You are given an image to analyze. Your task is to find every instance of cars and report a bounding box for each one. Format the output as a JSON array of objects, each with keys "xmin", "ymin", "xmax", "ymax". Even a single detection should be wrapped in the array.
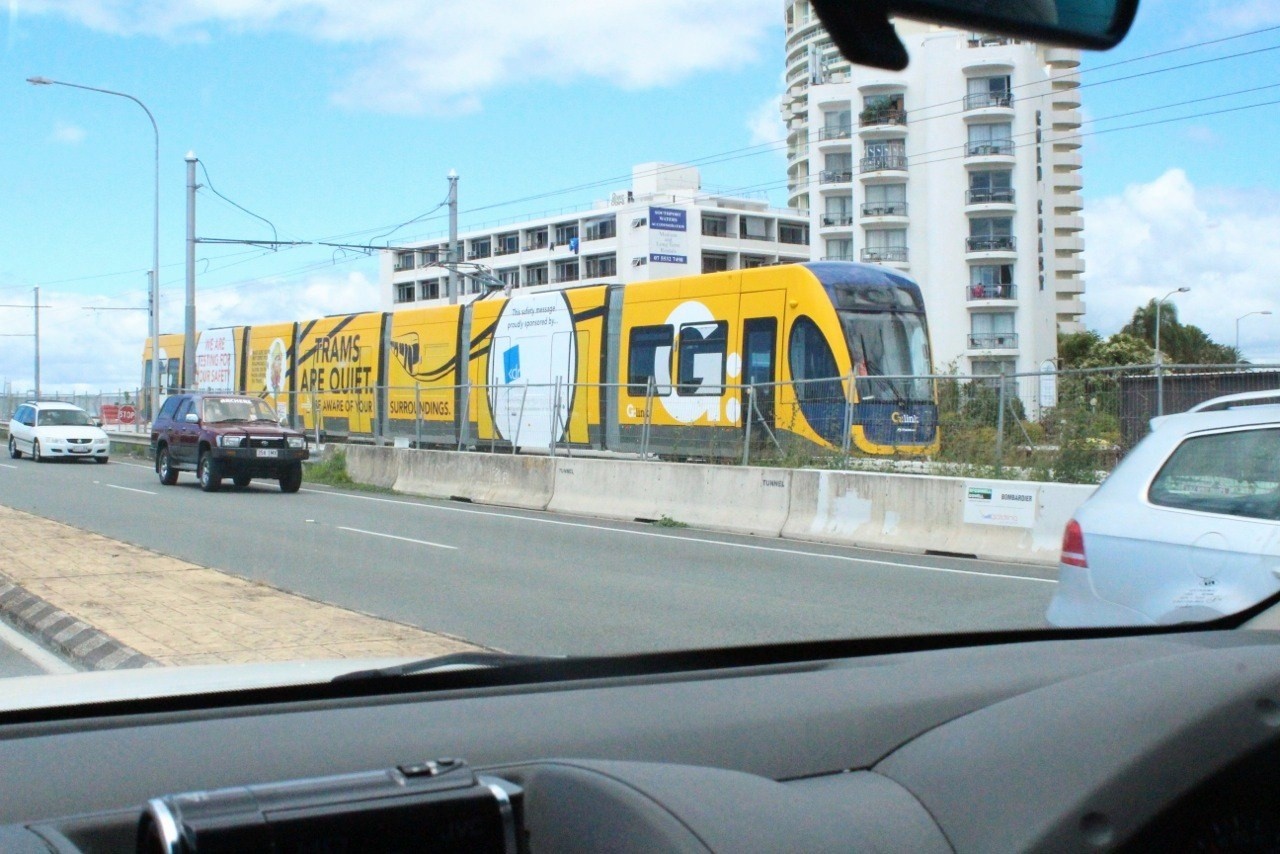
[
  {"xmin": 7, "ymin": 400, "xmax": 110, "ymax": 464},
  {"xmin": 1045, "ymin": 386, "xmax": 1280, "ymax": 627}
]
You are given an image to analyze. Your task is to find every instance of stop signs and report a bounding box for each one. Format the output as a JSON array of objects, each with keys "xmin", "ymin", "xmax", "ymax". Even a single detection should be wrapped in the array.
[{"xmin": 116, "ymin": 405, "xmax": 137, "ymax": 425}]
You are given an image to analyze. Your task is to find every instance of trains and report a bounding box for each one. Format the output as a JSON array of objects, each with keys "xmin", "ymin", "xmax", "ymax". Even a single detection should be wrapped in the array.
[{"xmin": 141, "ymin": 260, "xmax": 941, "ymax": 470}]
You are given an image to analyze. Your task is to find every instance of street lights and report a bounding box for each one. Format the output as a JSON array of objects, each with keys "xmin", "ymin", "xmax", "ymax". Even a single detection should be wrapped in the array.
[
  {"xmin": 1157, "ymin": 286, "xmax": 1191, "ymax": 416},
  {"xmin": 24, "ymin": 77, "xmax": 160, "ymax": 427},
  {"xmin": 1236, "ymin": 310, "xmax": 1273, "ymax": 372}
]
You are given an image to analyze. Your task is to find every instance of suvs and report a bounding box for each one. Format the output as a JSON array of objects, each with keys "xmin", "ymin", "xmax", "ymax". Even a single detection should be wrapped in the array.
[{"xmin": 147, "ymin": 393, "xmax": 308, "ymax": 493}]
